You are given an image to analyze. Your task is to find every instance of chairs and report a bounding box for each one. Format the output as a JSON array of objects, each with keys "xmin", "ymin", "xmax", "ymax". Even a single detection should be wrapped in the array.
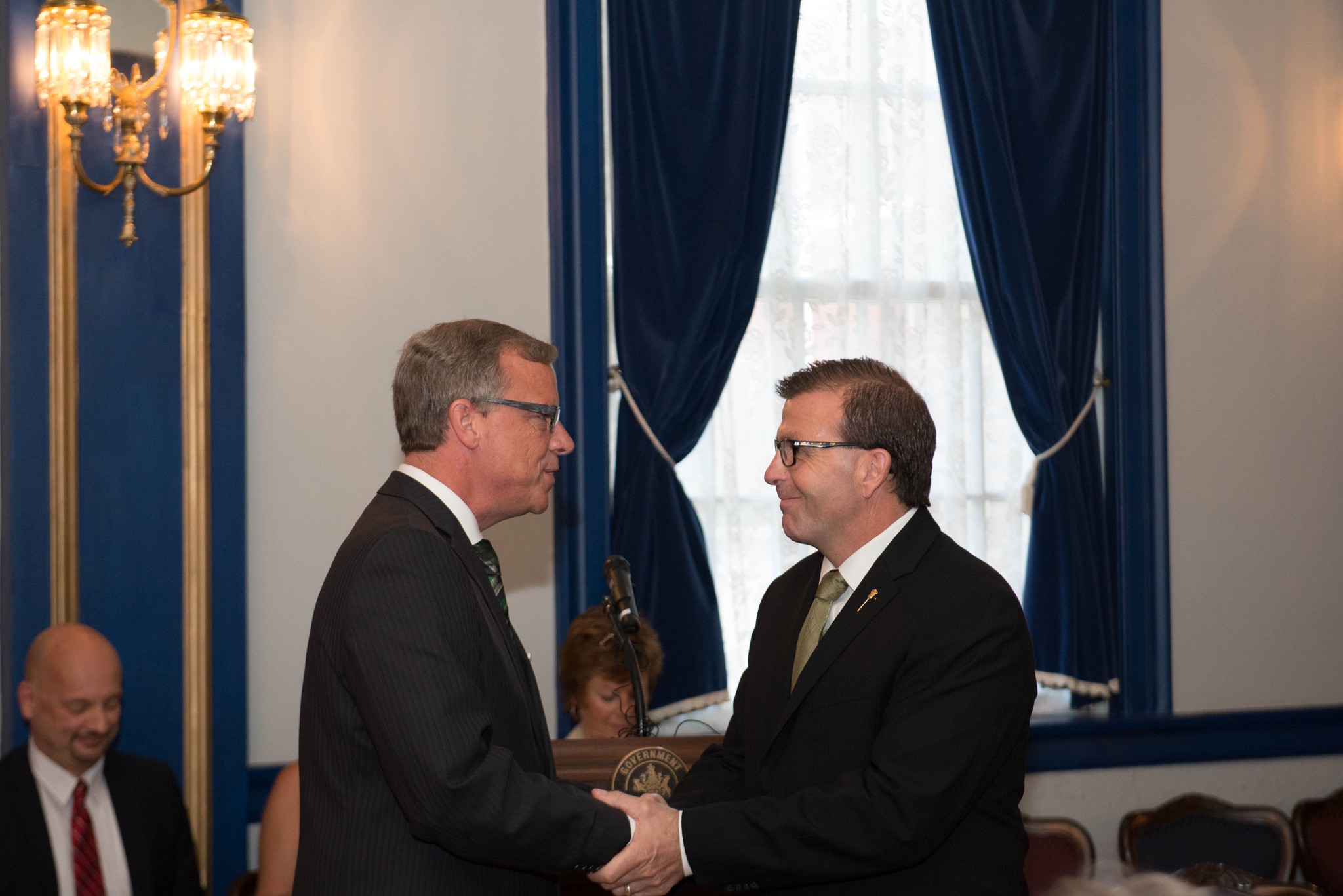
[
  {"xmin": 1289, "ymin": 787, "xmax": 1343, "ymax": 896},
  {"xmin": 1117, "ymin": 791, "xmax": 1298, "ymax": 884},
  {"xmin": 1020, "ymin": 811, "xmax": 1097, "ymax": 896}
]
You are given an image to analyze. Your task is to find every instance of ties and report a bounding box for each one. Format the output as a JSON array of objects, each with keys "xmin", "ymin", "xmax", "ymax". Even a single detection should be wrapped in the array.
[
  {"xmin": 789, "ymin": 569, "xmax": 848, "ymax": 695},
  {"xmin": 63, "ymin": 777, "xmax": 105, "ymax": 896},
  {"xmin": 474, "ymin": 539, "xmax": 509, "ymax": 621}
]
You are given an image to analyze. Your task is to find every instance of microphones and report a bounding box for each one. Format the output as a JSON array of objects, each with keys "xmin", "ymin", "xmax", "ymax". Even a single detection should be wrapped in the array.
[{"xmin": 605, "ymin": 555, "xmax": 642, "ymax": 635}]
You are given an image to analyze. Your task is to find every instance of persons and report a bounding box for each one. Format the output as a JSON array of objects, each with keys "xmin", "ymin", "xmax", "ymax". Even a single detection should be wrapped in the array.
[
  {"xmin": 289, "ymin": 318, "xmax": 659, "ymax": 896},
  {"xmin": 587, "ymin": 352, "xmax": 1037, "ymax": 896},
  {"xmin": 0, "ymin": 623, "xmax": 202, "ymax": 896},
  {"xmin": 559, "ymin": 601, "xmax": 663, "ymax": 738},
  {"xmin": 257, "ymin": 760, "xmax": 300, "ymax": 896}
]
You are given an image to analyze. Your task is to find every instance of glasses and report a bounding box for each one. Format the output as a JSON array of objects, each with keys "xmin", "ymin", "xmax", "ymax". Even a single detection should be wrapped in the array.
[
  {"xmin": 774, "ymin": 438, "xmax": 898, "ymax": 474},
  {"xmin": 469, "ymin": 397, "xmax": 561, "ymax": 434}
]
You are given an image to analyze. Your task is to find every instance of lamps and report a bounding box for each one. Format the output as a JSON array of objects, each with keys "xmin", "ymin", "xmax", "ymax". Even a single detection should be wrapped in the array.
[{"xmin": 31, "ymin": 0, "xmax": 262, "ymax": 247}]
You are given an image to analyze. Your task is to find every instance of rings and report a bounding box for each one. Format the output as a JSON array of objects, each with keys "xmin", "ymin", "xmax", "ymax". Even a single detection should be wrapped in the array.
[{"xmin": 625, "ymin": 883, "xmax": 631, "ymax": 896}]
